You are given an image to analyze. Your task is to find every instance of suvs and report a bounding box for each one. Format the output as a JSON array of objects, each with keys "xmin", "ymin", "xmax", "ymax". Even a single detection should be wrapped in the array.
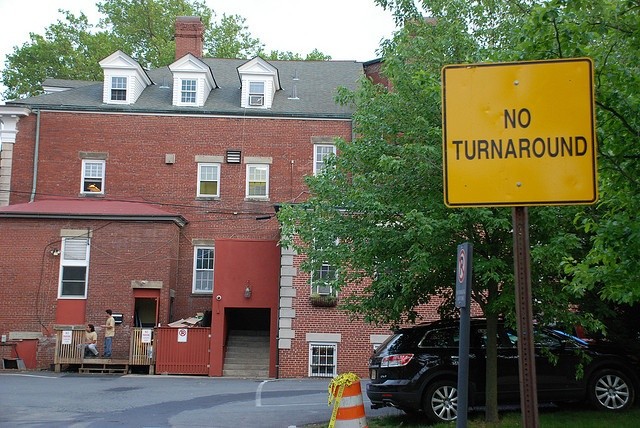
[{"xmin": 367, "ymin": 316, "xmax": 640, "ymax": 423}]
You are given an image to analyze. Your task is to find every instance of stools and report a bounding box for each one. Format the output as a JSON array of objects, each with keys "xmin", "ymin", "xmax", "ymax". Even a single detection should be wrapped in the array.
[{"xmin": 85, "ymin": 346, "xmax": 97, "ymax": 357}]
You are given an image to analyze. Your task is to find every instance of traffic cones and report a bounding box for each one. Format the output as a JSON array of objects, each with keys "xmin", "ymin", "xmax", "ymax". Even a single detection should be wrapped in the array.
[{"xmin": 332, "ymin": 380, "xmax": 369, "ymax": 428}]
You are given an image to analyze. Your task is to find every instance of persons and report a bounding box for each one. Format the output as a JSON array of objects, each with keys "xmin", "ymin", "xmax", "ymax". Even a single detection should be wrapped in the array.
[
  {"xmin": 100, "ymin": 308, "xmax": 115, "ymax": 358},
  {"xmin": 83, "ymin": 323, "xmax": 99, "ymax": 357}
]
